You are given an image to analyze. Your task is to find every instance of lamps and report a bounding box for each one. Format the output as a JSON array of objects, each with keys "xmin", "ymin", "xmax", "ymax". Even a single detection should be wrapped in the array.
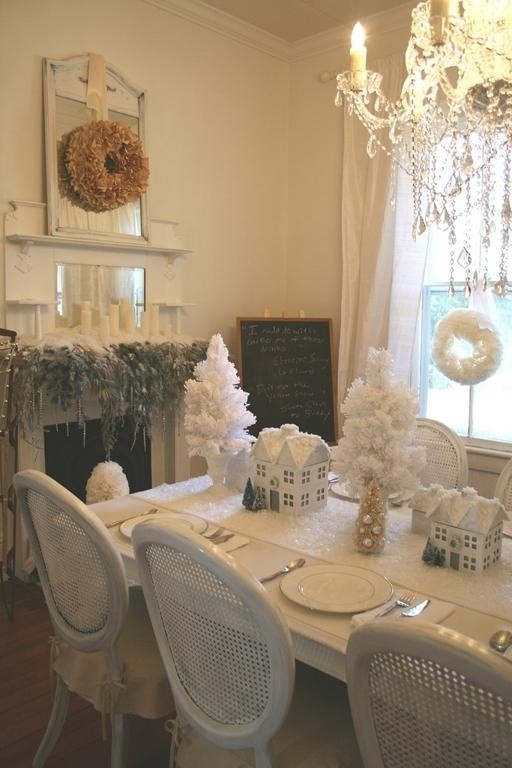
[{"xmin": 336, "ymin": 2, "xmax": 512, "ymax": 300}]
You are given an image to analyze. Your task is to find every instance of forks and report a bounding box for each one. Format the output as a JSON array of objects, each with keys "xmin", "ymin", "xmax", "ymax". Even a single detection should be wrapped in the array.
[
  {"xmin": 374, "ymin": 591, "xmax": 417, "ymax": 619},
  {"xmin": 202, "ymin": 526, "xmax": 226, "ymax": 539}
]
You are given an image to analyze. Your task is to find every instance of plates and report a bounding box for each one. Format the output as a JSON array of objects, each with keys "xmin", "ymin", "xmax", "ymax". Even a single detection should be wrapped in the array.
[
  {"xmin": 118, "ymin": 511, "xmax": 209, "ymax": 543},
  {"xmin": 279, "ymin": 563, "xmax": 394, "ymax": 614},
  {"xmin": 331, "ymin": 479, "xmax": 401, "ymax": 500}
]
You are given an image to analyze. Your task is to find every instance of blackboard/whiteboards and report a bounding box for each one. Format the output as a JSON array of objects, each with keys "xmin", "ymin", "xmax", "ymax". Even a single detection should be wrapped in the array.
[{"xmin": 237, "ymin": 317, "xmax": 338, "ymax": 446}]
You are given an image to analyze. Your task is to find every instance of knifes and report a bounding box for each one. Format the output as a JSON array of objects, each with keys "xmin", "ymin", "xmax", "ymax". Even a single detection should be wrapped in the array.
[
  {"xmin": 395, "ymin": 599, "xmax": 430, "ymax": 618},
  {"xmin": 209, "ymin": 534, "xmax": 235, "ymax": 545}
]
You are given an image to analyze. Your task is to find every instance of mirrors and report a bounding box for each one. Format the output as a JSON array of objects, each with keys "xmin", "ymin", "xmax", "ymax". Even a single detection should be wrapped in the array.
[
  {"xmin": 42, "ymin": 54, "xmax": 152, "ymax": 241},
  {"xmin": 56, "ymin": 262, "xmax": 145, "ymax": 329}
]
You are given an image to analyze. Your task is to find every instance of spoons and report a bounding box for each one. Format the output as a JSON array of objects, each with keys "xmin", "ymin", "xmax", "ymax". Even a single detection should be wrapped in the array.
[
  {"xmin": 489, "ymin": 629, "xmax": 512, "ymax": 654},
  {"xmin": 390, "ymin": 495, "xmax": 412, "ymax": 507},
  {"xmin": 257, "ymin": 558, "xmax": 306, "ymax": 585},
  {"xmin": 104, "ymin": 508, "xmax": 158, "ymax": 529}
]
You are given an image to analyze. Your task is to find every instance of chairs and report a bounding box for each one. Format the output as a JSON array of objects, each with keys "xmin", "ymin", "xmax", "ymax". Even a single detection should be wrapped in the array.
[
  {"xmin": 491, "ymin": 454, "xmax": 511, "ymax": 510},
  {"xmin": 414, "ymin": 419, "xmax": 469, "ymax": 492},
  {"xmin": 125, "ymin": 515, "xmax": 296, "ymax": 766},
  {"xmin": 12, "ymin": 467, "xmax": 173, "ymax": 765},
  {"xmin": 335, "ymin": 616, "xmax": 512, "ymax": 767}
]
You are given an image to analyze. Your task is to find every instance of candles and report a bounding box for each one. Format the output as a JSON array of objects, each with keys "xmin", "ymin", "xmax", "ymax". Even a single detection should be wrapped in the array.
[{"xmin": 78, "ymin": 297, "xmax": 182, "ymax": 343}]
